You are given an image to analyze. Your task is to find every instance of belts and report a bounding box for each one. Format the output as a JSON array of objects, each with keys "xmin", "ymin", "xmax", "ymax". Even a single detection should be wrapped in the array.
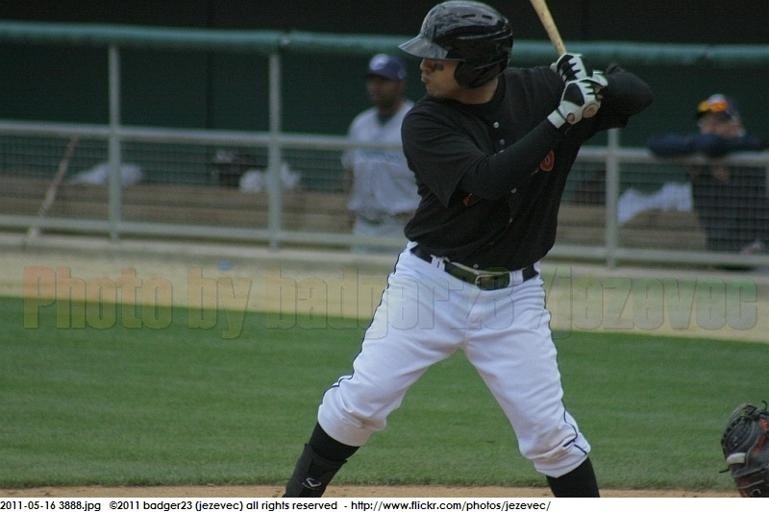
[{"xmin": 410, "ymin": 241, "xmax": 539, "ymax": 290}]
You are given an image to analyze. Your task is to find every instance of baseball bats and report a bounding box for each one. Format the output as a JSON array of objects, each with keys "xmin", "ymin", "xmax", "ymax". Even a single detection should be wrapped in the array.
[{"xmin": 532, "ymin": 1, "xmax": 599, "ymax": 118}]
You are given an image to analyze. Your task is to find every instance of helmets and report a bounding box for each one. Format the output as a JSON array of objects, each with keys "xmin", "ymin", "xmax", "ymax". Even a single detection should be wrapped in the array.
[{"xmin": 398, "ymin": 0, "xmax": 514, "ymax": 90}]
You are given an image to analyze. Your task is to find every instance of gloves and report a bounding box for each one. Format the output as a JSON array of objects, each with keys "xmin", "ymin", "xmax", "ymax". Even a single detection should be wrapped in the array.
[{"xmin": 546, "ymin": 51, "xmax": 609, "ymax": 135}]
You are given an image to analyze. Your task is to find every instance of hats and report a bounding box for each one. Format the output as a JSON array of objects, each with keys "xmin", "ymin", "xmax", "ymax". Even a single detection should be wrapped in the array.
[{"xmin": 364, "ymin": 53, "xmax": 405, "ymax": 80}]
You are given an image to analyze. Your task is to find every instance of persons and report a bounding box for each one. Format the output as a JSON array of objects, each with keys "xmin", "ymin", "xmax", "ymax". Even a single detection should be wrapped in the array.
[
  {"xmin": 646, "ymin": 94, "xmax": 769, "ymax": 272},
  {"xmin": 281, "ymin": 0, "xmax": 657, "ymax": 497},
  {"xmin": 341, "ymin": 52, "xmax": 422, "ymax": 257}
]
